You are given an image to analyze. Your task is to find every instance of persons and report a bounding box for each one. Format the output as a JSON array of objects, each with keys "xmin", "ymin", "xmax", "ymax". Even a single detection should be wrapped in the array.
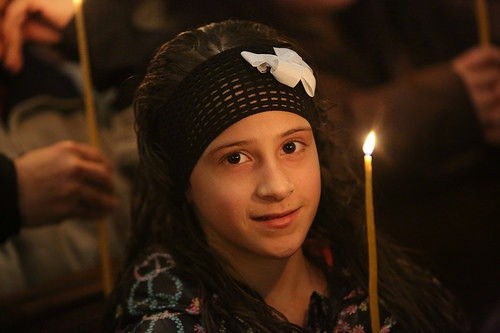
[
  {"xmin": 110, "ymin": 17, "xmax": 466, "ymax": 333},
  {"xmin": 277, "ymin": 1, "xmax": 500, "ymax": 332}
]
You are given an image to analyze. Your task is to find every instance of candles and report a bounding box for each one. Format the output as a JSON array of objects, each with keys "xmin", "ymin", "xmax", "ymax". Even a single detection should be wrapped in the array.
[
  {"xmin": 70, "ymin": 0, "xmax": 118, "ymax": 296},
  {"xmin": 361, "ymin": 128, "xmax": 383, "ymax": 332}
]
